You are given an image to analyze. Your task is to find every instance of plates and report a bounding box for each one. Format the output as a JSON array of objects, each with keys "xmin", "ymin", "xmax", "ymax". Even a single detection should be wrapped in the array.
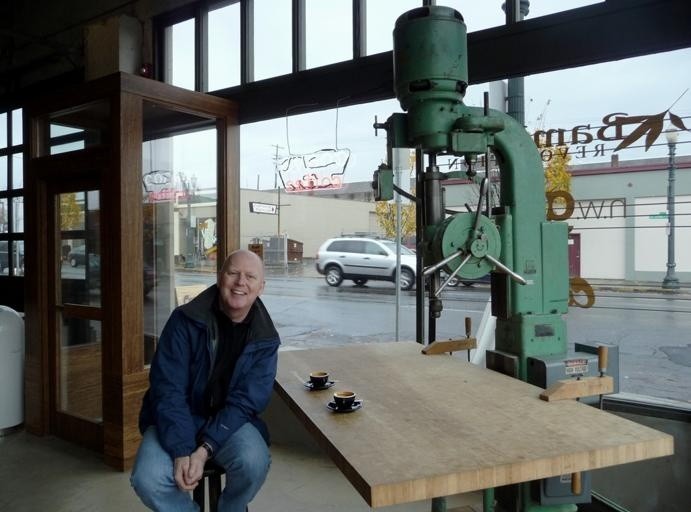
[
  {"xmin": 303, "ymin": 379, "xmax": 336, "ymax": 390},
  {"xmin": 327, "ymin": 398, "xmax": 363, "ymax": 414}
]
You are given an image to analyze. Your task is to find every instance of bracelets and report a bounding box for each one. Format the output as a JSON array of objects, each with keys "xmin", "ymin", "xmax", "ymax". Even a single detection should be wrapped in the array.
[{"xmin": 200, "ymin": 438, "xmax": 213, "ymax": 460}]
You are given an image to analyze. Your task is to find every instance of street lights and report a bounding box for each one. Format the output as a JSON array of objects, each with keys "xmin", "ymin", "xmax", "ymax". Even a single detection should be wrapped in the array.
[{"xmin": 663, "ymin": 123, "xmax": 681, "ymax": 289}]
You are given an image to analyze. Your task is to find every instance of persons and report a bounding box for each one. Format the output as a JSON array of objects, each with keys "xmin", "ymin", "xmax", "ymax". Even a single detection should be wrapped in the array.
[{"xmin": 129, "ymin": 247, "xmax": 280, "ymax": 512}]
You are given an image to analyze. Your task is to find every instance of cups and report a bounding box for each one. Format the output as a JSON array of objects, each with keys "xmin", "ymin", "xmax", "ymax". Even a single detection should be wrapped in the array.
[
  {"xmin": 334, "ymin": 390, "xmax": 356, "ymax": 409},
  {"xmin": 310, "ymin": 370, "xmax": 330, "ymax": 387}
]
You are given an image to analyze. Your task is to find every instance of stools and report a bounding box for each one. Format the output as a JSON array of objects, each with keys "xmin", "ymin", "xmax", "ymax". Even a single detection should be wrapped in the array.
[{"xmin": 192, "ymin": 460, "xmax": 227, "ymax": 511}]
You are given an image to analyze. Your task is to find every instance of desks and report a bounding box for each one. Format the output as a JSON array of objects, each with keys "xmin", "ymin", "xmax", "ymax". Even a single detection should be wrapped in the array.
[{"xmin": 273, "ymin": 341, "xmax": 675, "ymax": 507}]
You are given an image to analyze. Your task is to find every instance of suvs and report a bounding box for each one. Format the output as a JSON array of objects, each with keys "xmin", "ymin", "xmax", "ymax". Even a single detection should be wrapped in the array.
[{"xmin": 316, "ymin": 237, "xmax": 417, "ymax": 290}]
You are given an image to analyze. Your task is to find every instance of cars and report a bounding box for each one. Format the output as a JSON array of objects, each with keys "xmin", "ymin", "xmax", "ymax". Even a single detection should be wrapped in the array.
[{"xmin": 67, "ymin": 245, "xmax": 86, "ymax": 267}]
